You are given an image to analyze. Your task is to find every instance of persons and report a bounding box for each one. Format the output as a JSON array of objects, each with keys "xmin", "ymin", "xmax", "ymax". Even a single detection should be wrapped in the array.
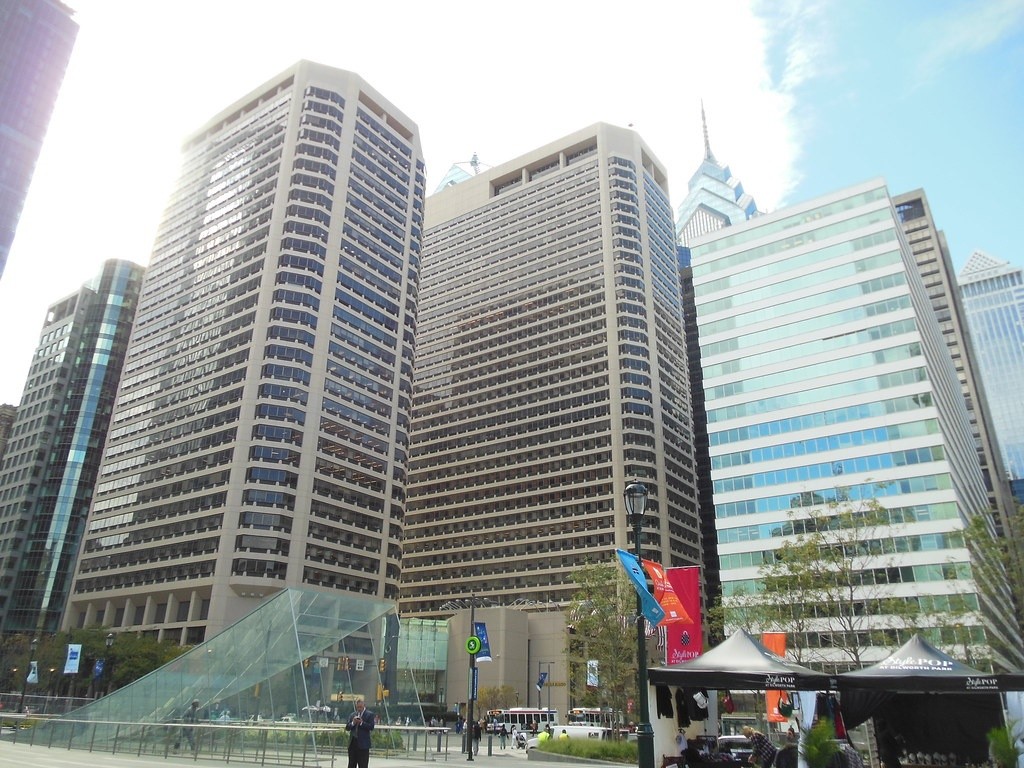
[
  {"xmin": 742, "ymin": 725, "xmax": 777, "ymax": 768},
  {"xmin": 373, "ymin": 713, "xmax": 449, "ymax": 734},
  {"xmin": 455, "ymin": 714, "xmax": 571, "ymax": 756},
  {"xmin": 345, "ymin": 699, "xmax": 375, "ymax": 768},
  {"xmin": 247, "ymin": 715, "xmax": 266, "ymax": 726},
  {"xmin": 175, "ymin": 699, "xmax": 199, "ymax": 756},
  {"xmin": 220, "ymin": 710, "xmax": 230, "ymax": 725}
]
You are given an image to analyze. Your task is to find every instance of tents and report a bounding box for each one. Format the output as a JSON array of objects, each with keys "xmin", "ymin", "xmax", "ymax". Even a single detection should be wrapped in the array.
[
  {"xmin": 837, "ymin": 633, "xmax": 1024, "ymax": 768},
  {"xmin": 648, "ymin": 628, "xmax": 834, "ymax": 768}
]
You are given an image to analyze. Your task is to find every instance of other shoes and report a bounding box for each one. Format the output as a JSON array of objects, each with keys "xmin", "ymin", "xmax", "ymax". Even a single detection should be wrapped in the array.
[
  {"xmin": 173, "ymin": 748, "xmax": 179, "ymax": 754},
  {"xmin": 190, "ymin": 750, "xmax": 197, "ymax": 755}
]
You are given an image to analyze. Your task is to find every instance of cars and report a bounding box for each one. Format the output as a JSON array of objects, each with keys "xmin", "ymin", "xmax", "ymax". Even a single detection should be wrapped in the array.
[{"xmin": 718, "ymin": 735, "xmax": 782, "ymax": 753}]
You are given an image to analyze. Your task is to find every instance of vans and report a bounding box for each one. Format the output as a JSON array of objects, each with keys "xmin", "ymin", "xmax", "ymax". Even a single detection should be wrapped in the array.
[{"xmin": 525, "ymin": 726, "xmax": 629, "ymax": 757}]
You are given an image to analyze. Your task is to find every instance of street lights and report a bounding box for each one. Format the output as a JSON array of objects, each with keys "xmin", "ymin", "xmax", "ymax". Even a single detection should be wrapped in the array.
[
  {"xmin": 466, "ymin": 594, "xmax": 485, "ymax": 761},
  {"xmin": 624, "ymin": 478, "xmax": 655, "ymax": 768},
  {"xmin": 204, "ymin": 649, "xmax": 212, "ymax": 719},
  {"xmin": 42, "ymin": 669, "xmax": 55, "ymax": 714},
  {"xmin": 103, "ymin": 633, "xmax": 115, "ymax": 696},
  {"xmin": 538, "ymin": 660, "xmax": 555, "ymax": 707},
  {"xmin": 10, "ymin": 638, "xmax": 39, "ymax": 731},
  {"xmin": 5, "ymin": 669, "xmax": 17, "ymax": 712},
  {"xmin": 440, "ymin": 688, "xmax": 443, "ymax": 704}
]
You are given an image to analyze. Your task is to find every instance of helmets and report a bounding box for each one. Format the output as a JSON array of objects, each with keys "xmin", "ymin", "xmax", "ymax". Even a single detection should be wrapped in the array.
[{"xmin": 191, "ymin": 699, "xmax": 199, "ymax": 705}]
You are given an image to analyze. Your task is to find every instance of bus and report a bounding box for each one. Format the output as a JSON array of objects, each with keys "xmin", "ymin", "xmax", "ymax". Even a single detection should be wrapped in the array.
[
  {"xmin": 484, "ymin": 707, "xmax": 559, "ymax": 734},
  {"xmin": 565, "ymin": 707, "xmax": 624, "ymax": 728}
]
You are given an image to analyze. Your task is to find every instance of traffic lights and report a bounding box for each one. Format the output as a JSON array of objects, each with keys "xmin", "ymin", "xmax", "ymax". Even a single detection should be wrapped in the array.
[
  {"xmin": 304, "ymin": 659, "xmax": 309, "ymax": 669},
  {"xmin": 380, "ymin": 660, "xmax": 385, "ymax": 672},
  {"xmin": 337, "ymin": 657, "xmax": 349, "ymax": 671}
]
